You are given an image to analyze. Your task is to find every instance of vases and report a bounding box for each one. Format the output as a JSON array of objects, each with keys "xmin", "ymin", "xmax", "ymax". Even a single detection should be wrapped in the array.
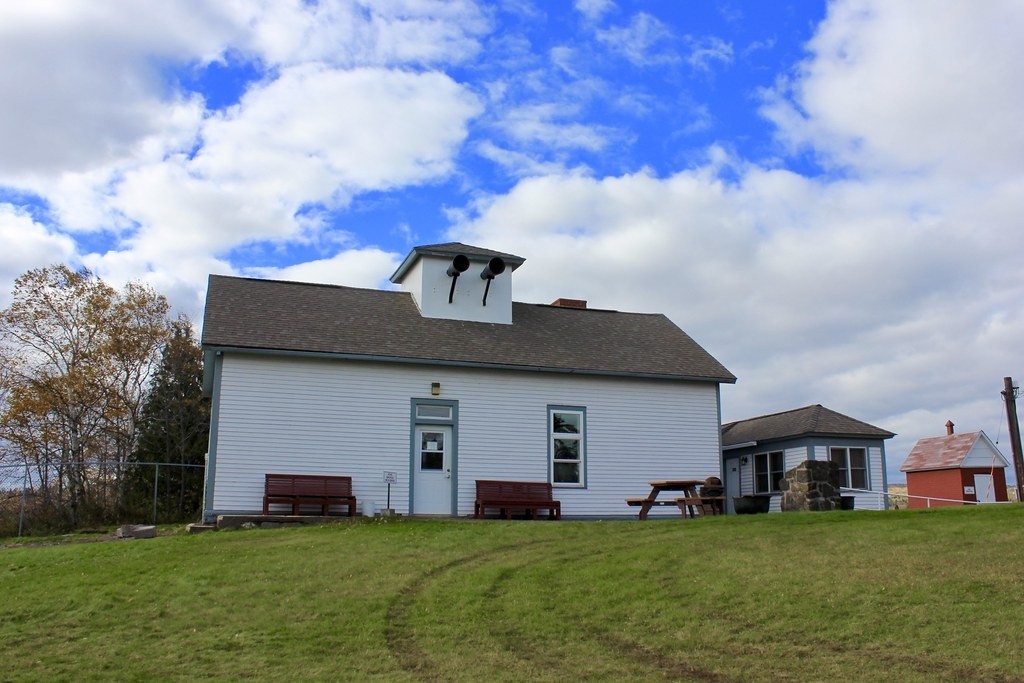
[
  {"xmin": 732, "ymin": 494, "xmax": 773, "ymax": 514},
  {"xmin": 841, "ymin": 496, "xmax": 856, "ymax": 510}
]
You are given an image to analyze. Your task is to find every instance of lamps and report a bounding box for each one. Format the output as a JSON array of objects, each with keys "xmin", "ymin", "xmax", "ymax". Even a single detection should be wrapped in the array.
[
  {"xmin": 742, "ymin": 456, "xmax": 748, "ymax": 465},
  {"xmin": 432, "ymin": 383, "xmax": 440, "ymax": 395}
]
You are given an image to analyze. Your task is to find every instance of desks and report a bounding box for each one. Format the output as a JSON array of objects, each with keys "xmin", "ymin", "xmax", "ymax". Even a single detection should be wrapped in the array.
[{"xmin": 639, "ymin": 481, "xmax": 723, "ymax": 520}]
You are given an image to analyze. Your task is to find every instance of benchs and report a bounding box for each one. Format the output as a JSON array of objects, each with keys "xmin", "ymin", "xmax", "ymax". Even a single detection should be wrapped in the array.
[
  {"xmin": 263, "ymin": 474, "xmax": 356, "ymax": 517},
  {"xmin": 475, "ymin": 480, "xmax": 561, "ymax": 520},
  {"xmin": 674, "ymin": 496, "xmax": 727, "ymax": 506},
  {"xmin": 625, "ymin": 498, "xmax": 685, "ymax": 506}
]
